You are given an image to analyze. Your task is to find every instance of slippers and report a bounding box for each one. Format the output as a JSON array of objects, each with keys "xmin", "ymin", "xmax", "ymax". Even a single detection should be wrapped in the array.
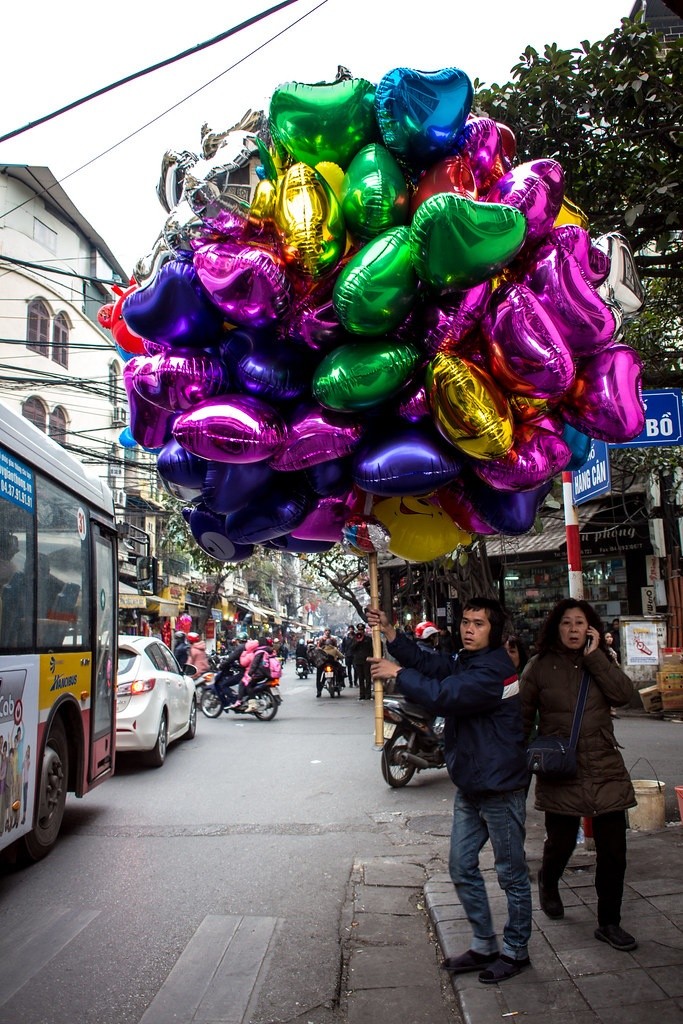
[
  {"xmin": 439, "ymin": 949, "xmax": 501, "ymax": 971},
  {"xmin": 478, "ymin": 956, "xmax": 533, "ymax": 983}
]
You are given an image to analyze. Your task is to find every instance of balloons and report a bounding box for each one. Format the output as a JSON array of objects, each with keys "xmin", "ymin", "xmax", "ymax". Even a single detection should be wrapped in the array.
[{"xmin": 97, "ymin": 66, "xmax": 651, "ymax": 562}]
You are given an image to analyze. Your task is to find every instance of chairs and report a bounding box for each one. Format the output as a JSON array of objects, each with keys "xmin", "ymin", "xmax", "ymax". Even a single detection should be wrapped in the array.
[
  {"xmin": 38, "ymin": 580, "xmax": 80, "ymax": 650},
  {"xmin": 0, "ymin": 573, "xmax": 28, "ymax": 654}
]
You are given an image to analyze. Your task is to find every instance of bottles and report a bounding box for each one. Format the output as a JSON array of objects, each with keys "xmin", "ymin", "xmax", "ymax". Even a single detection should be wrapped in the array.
[{"xmin": 503, "ymin": 560, "xmax": 618, "ymax": 619}]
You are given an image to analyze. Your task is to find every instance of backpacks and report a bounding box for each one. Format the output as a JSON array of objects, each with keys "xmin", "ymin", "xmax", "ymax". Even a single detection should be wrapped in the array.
[{"xmin": 264, "ymin": 652, "xmax": 281, "ymax": 680}]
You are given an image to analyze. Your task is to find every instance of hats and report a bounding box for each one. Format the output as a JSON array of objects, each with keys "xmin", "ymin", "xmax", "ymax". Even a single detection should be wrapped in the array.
[
  {"xmin": 273, "ymin": 637, "xmax": 281, "ymax": 643},
  {"xmin": 299, "ymin": 639, "xmax": 304, "ymax": 645},
  {"xmin": 356, "ymin": 623, "xmax": 366, "ymax": 630}
]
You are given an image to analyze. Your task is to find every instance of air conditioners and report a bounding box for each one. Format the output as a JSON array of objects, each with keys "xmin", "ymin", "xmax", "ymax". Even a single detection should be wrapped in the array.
[
  {"xmin": 113, "ymin": 406, "xmax": 130, "ymax": 424},
  {"xmin": 114, "ymin": 489, "xmax": 127, "ymax": 506}
]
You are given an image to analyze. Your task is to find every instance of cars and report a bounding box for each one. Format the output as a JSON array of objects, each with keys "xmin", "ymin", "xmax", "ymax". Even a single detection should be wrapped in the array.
[{"xmin": 62, "ymin": 634, "xmax": 198, "ymax": 768}]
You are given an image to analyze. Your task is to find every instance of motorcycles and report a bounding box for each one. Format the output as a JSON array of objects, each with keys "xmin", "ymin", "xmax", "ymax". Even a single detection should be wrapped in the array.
[{"xmin": 168, "ymin": 645, "xmax": 286, "ymax": 722}]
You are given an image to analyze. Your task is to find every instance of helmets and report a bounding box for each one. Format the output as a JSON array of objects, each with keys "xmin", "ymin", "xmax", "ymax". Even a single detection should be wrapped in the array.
[
  {"xmin": 174, "ymin": 631, "xmax": 186, "ymax": 642},
  {"xmin": 186, "ymin": 631, "xmax": 201, "ymax": 643},
  {"xmin": 414, "ymin": 621, "xmax": 441, "ymax": 640},
  {"xmin": 236, "ymin": 631, "xmax": 249, "ymax": 641}
]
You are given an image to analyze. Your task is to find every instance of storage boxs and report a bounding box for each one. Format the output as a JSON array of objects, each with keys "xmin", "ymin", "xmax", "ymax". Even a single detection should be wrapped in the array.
[{"xmin": 639, "ymin": 648, "xmax": 683, "ymax": 714}]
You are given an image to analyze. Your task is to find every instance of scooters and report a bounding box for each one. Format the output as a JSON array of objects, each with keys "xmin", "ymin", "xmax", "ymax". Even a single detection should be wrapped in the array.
[
  {"xmin": 295, "ymin": 656, "xmax": 314, "ymax": 680},
  {"xmin": 373, "ymin": 694, "xmax": 447, "ymax": 789},
  {"xmin": 319, "ymin": 656, "xmax": 346, "ymax": 698}
]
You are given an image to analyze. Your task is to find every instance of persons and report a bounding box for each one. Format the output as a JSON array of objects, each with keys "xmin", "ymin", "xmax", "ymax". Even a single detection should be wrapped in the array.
[
  {"xmin": 186, "ymin": 631, "xmax": 211, "ymax": 679},
  {"xmin": 18, "ymin": 550, "xmax": 78, "ymax": 617},
  {"xmin": 306, "ymin": 639, "xmax": 317, "ymax": 653},
  {"xmin": 315, "ymin": 627, "xmax": 345, "ymax": 698},
  {"xmin": 216, "ymin": 631, "xmax": 274, "ymax": 712},
  {"xmin": 0, "ymin": 526, "xmax": 24, "ymax": 650},
  {"xmin": 296, "ymin": 638, "xmax": 311, "ymax": 670},
  {"xmin": 501, "ymin": 634, "xmax": 533, "ymax": 859},
  {"xmin": 603, "ymin": 631, "xmax": 620, "ymax": 666},
  {"xmin": 365, "ymin": 598, "xmax": 532, "ymax": 983},
  {"xmin": 274, "ymin": 637, "xmax": 288, "ymax": 669},
  {"xmin": 341, "ymin": 623, "xmax": 383, "ymax": 687},
  {"xmin": 414, "ymin": 622, "xmax": 445, "ymax": 745},
  {"xmin": 173, "ymin": 631, "xmax": 190, "ymax": 668},
  {"xmin": 611, "ymin": 619, "xmax": 621, "ymax": 652},
  {"xmin": 518, "ymin": 597, "xmax": 639, "ymax": 950},
  {"xmin": 437, "ymin": 622, "xmax": 456, "ymax": 654},
  {"xmin": 403, "ymin": 624, "xmax": 413, "ymax": 640}
]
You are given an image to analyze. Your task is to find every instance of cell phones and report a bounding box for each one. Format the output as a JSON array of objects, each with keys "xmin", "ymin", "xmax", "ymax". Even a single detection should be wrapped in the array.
[{"xmin": 587, "ymin": 636, "xmax": 592, "ymax": 648}]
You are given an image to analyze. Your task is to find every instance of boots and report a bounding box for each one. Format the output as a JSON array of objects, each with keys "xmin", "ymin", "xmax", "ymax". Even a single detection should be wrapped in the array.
[{"xmin": 244, "ymin": 698, "xmax": 258, "ymax": 713}]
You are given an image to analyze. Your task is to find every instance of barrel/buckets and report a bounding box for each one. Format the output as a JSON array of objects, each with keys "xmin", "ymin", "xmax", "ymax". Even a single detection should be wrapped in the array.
[
  {"xmin": 627, "ymin": 757, "xmax": 667, "ymax": 834},
  {"xmin": 674, "ymin": 786, "xmax": 683, "ymax": 825},
  {"xmin": 660, "ymin": 647, "xmax": 683, "ymax": 672}
]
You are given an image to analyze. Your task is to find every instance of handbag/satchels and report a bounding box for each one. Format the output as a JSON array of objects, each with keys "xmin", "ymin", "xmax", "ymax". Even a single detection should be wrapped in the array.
[{"xmin": 524, "ymin": 739, "xmax": 576, "ymax": 780}]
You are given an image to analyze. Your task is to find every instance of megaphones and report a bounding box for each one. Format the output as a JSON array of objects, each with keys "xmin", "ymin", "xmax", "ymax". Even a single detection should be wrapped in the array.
[{"xmin": 355, "ymin": 632, "xmax": 364, "ymax": 641}]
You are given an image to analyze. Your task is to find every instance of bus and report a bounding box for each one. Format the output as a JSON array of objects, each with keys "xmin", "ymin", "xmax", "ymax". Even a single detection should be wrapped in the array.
[{"xmin": 0, "ymin": 400, "xmax": 158, "ymax": 868}]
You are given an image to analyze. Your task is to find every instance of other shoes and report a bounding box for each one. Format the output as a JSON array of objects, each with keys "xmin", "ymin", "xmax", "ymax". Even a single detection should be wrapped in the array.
[
  {"xmin": 537, "ymin": 868, "xmax": 564, "ymax": 919},
  {"xmin": 222, "ymin": 702, "xmax": 235, "ymax": 709},
  {"xmin": 594, "ymin": 922, "xmax": 638, "ymax": 952},
  {"xmin": 231, "ymin": 699, "xmax": 243, "ymax": 708}
]
